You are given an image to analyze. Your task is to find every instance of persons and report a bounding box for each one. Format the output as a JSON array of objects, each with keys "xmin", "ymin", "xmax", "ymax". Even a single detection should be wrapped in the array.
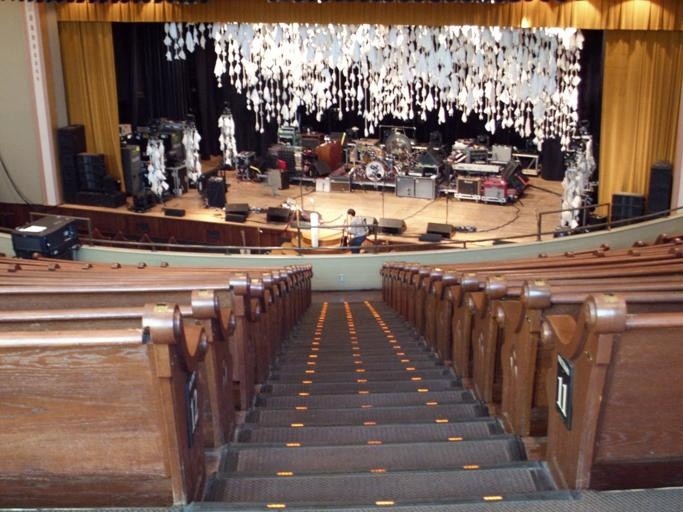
[{"xmin": 347, "ymin": 209, "xmax": 369, "ymax": 254}]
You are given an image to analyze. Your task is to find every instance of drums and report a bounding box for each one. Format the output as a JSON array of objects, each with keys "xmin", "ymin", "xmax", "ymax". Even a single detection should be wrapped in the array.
[{"xmin": 364, "ymin": 160, "xmax": 386, "ymax": 182}]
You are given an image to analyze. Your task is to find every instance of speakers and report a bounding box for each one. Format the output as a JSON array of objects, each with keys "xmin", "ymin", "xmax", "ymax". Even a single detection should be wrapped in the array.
[
  {"xmin": 208, "ymin": 177, "xmax": 227, "ymax": 208},
  {"xmin": 612, "ymin": 193, "xmax": 645, "ymax": 229},
  {"xmin": 647, "ymin": 161, "xmax": 674, "ymax": 222},
  {"xmin": 77, "ymin": 152, "xmax": 106, "ymax": 193},
  {"xmin": 55, "ymin": 123, "xmax": 87, "ymax": 189}
]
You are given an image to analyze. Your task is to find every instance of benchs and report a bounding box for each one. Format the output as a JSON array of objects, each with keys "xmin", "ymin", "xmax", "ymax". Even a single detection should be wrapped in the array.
[
  {"xmin": 306, "ymin": 265, "xmax": 311, "ymax": 307},
  {"xmin": 432, "ymin": 275, "xmax": 683, "ymax": 379},
  {"xmin": 279, "ymin": 270, "xmax": 291, "ymax": 339},
  {"xmin": 290, "ymin": 265, "xmax": 301, "ymax": 320},
  {"xmin": 467, "ymin": 277, "xmax": 682, "ymax": 402},
  {"xmin": 380, "ymin": 263, "xmax": 386, "ymax": 301},
  {"xmin": 387, "ymin": 262, "xmax": 393, "ymax": 306},
  {"xmin": 494, "ymin": 278, "xmax": 682, "ymax": 437},
  {"xmin": 0, "ymin": 304, "xmax": 209, "ymax": 507},
  {"xmin": 412, "ymin": 266, "xmax": 429, "ymax": 337},
  {"xmin": 303, "ymin": 265, "xmax": 308, "ymax": 311},
  {"xmin": 423, "ymin": 268, "xmax": 444, "ymax": 352},
  {"xmin": 391, "ymin": 264, "xmax": 398, "ymax": 310},
  {"xmin": 406, "ymin": 263, "xmax": 419, "ymax": 327},
  {"xmin": 439, "ymin": 273, "xmax": 457, "ymax": 362},
  {"xmin": 539, "ymin": 293, "xmax": 683, "ymax": 490},
  {"xmin": 394, "ymin": 263, "xmax": 404, "ymax": 314},
  {"xmin": 0, "ymin": 280, "xmax": 271, "ymax": 383},
  {"xmin": 309, "ymin": 265, "xmax": 313, "ymax": 304},
  {"xmin": 399, "ymin": 265, "xmax": 412, "ymax": 321},
  {"xmin": 0, "ymin": 290, "xmax": 235, "ymax": 448},
  {"xmin": 385, "ymin": 263, "xmax": 390, "ymax": 302},
  {"xmin": 300, "ymin": 266, "xmax": 306, "ymax": 312},
  {"xmin": 0, "ymin": 272, "xmax": 261, "ymax": 410},
  {"xmin": 285, "ymin": 267, "xmax": 297, "ymax": 326},
  {"xmin": 295, "ymin": 265, "xmax": 302, "ymax": 313}
]
